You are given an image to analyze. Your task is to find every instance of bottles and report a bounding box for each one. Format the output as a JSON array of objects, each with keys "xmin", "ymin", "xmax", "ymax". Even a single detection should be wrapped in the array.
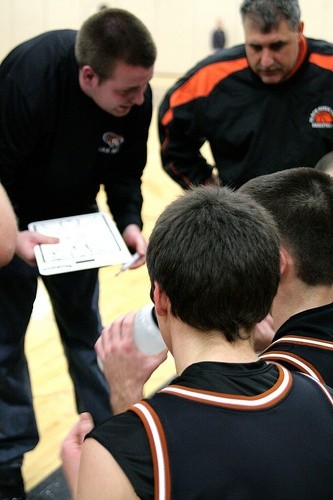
[{"xmin": 96, "ymin": 303, "xmax": 168, "ymax": 374}]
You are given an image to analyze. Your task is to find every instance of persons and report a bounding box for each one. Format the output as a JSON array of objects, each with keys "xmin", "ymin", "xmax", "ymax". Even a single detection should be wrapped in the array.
[
  {"xmin": 0, "ymin": 183, "xmax": 17, "ymax": 266},
  {"xmin": 61, "ymin": 184, "xmax": 333, "ymax": 500},
  {"xmin": 157, "ymin": 0, "xmax": 333, "ymax": 194},
  {"xmin": 95, "ymin": 166, "xmax": 333, "ymax": 416},
  {"xmin": 0, "ymin": 9, "xmax": 157, "ymax": 500}
]
